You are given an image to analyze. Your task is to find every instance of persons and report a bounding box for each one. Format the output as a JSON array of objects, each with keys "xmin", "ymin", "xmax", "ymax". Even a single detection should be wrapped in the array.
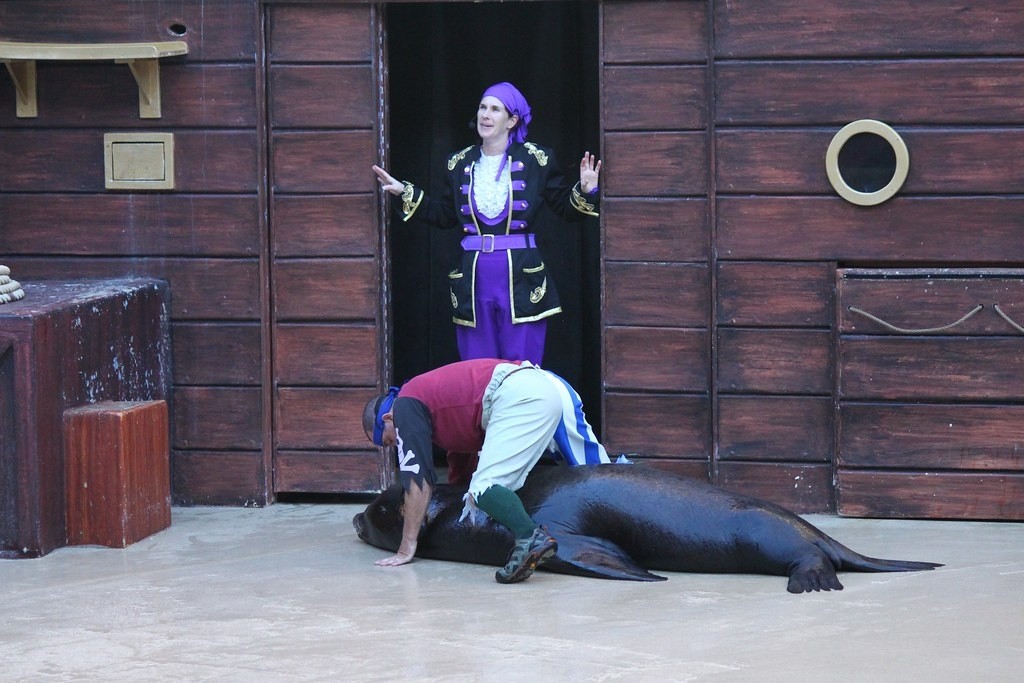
[
  {"xmin": 371, "ymin": 81, "xmax": 602, "ymax": 372},
  {"xmin": 362, "ymin": 357, "xmax": 611, "ymax": 584}
]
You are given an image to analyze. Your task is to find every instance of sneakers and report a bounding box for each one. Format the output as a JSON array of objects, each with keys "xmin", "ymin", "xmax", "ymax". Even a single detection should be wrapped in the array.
[{"xmin": 495, "ymin": 524, "xmax": 558, "ymax": 583}]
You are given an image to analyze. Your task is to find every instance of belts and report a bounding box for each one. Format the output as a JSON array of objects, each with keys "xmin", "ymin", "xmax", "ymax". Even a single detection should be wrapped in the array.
[{"xmin": 460, "ymin": 232, "xmax": 536, "ymax": 253}]
